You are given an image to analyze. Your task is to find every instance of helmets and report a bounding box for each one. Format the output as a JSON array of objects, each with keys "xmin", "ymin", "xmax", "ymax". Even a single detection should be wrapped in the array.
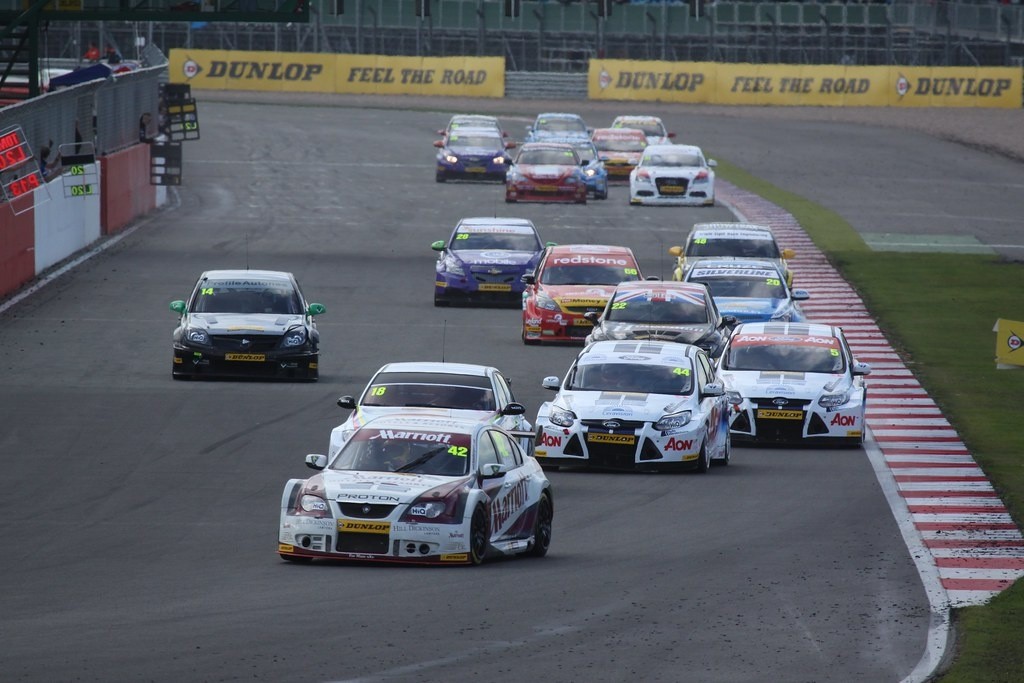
[
  {"xmin": 381, "ymin": 440, "xmax": 411, "ymax": 465},
  {"xmin": 600, "ymin": 363, "xmax": 633, "ymax": 386}
]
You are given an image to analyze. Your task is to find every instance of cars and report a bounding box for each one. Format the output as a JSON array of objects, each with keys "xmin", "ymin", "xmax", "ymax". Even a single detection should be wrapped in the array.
[
  {"xmin": 667, "ymin": 221, "xmax": 797, "ymax": 290},
  {"xmin": 629, "ymin": 145, "xmax": 718, "ymax": 207},
  {"xmin": 683, "ymin": 259, "xmax": 810, "ymax": 324},
  {"xmin": 433, "ymin": 127, "xmax": 517, "ymax": 184},
  {"xmin": 519, "ymin": 243, "xmax": 660, "ymax": 345},
  {"xmin": 169, "ymin": 270, "xmax": 328, "ymax": 382},
  {"xmin": 431, "ymin": 217, "xmax": 559, "ymax": 306},
  {"xmin": 524, "ymin": 137, "xmax": 610, "ymax": 200},
  {"xmin": 590, "ymin": 128, "xmax": 650, "ymax": 181},
  {"xmin": 525, "ymin": 112, "xmax": 594, "ymax": 141},
  {"xmin": 437, "ymin": 113, "xmax": 510, "ymax": 146},
  {"xmin": 532, "ymin": 341, "xmax": 731, "ymax": 473},
  {"xmin": 277, "ymin": 415, "xmax": 553, "ymax": 567},
  {"xmin": 708, "ymin": 322, "xmax": 872, "ymax": 449},
  {"xmin": 326, "ymin": 362, "xmax": 537, "ymax": 468},
  {"xmin": 583, "ymin": 280, "xmax": 737, "ymax": 372},
  {"xmin": 610, "ymin": 115, "xmax": 676, "ymax": 146},
  {"xmin": 503, "ymin": 143, "xmax": 589, "ymax": 204}
]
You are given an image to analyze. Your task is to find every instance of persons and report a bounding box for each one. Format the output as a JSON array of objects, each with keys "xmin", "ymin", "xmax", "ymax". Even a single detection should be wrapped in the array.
[
  {"xmin": 255, "ymin": 288, "xmax": 280, "ymax": 314},
  {"xmin": 82, "ymin": 40, "xmax": 121, "ymax": 74},
  {"xmin": 379, "ymin": 439, "xmax": 424, "ymax": 474},
  {"xmin": 39, "ymin": 145, "xmax": 61, "ymax": 178},
  {"xmin": 800, "ymin": 352, "xmax": 824, "ymax": 371},
  {"xmin": 426, "ymin": 383, "xmax": 483, "ymax": 408},
  {"xmin": 586, "ymin": 363, "xmax": 641, "ymax": 391}
]
